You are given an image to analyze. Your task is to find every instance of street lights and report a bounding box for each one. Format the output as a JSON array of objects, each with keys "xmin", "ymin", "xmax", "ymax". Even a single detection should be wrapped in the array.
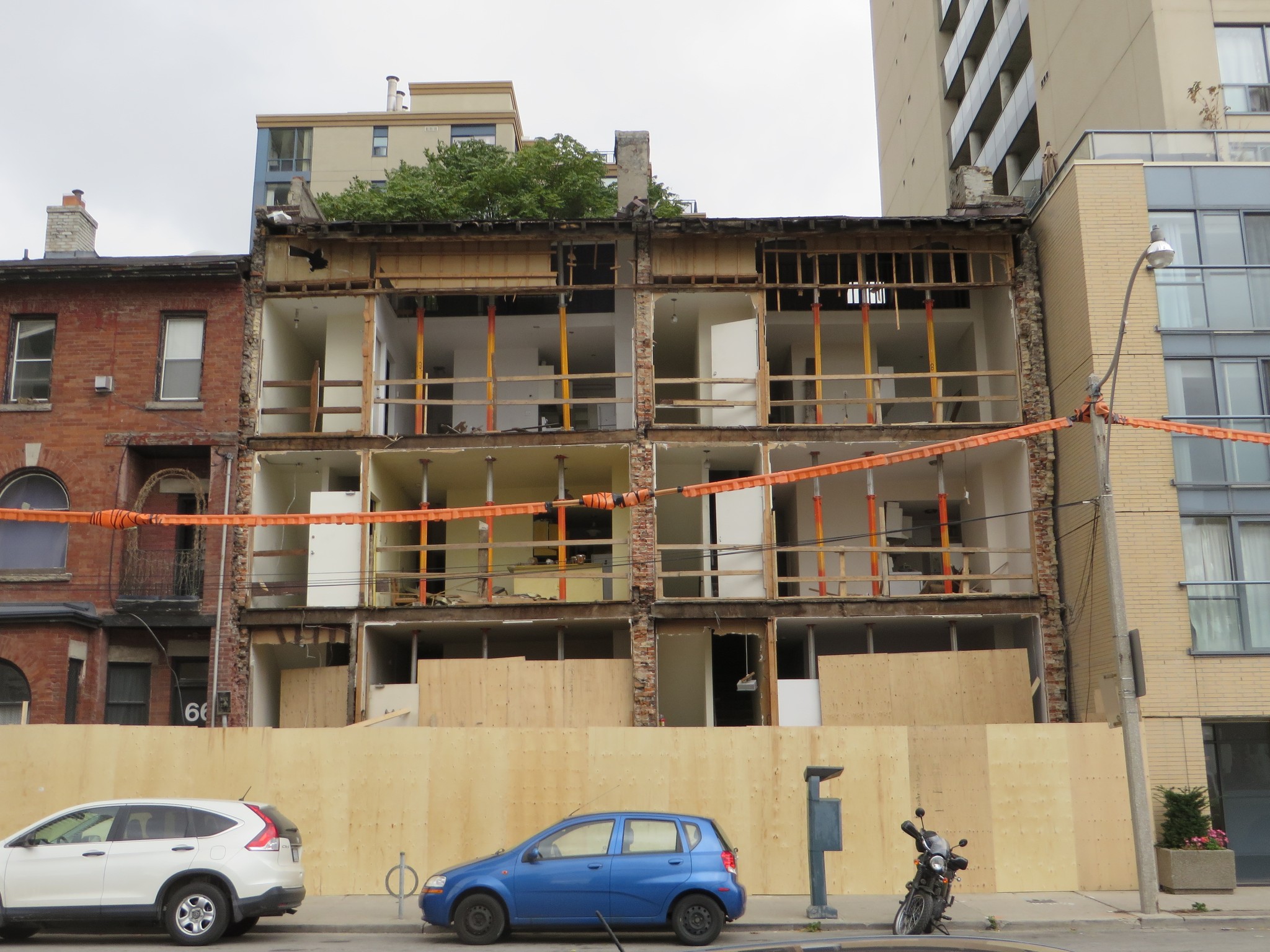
[{"xmin": 1089, "ymin": 222, "xmax": 1176, "ymax": 911}]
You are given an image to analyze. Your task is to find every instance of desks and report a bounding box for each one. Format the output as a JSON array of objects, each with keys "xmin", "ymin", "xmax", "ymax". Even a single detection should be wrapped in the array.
[{"xmin": 506, "ymin": 563, "xmax": 603, "ymax": 601}]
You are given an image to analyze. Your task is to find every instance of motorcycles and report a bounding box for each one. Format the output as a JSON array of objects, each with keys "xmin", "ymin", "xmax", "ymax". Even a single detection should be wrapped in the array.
[{"xmin": 895, "ymin": 807, "xmax": 970, "ymax": 936}]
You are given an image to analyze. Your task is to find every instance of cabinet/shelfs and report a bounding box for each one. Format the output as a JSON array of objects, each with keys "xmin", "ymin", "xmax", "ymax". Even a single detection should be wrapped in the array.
[
  {"xmin": 878, "ymin": 364, "xmax": 896, "ymax": 420},
  {"xmin": 889, "ymin": 571, "xmax": 923, "ymax": 597},
  {"xmin": 885, "ymin": 502, "xmax": 961, "ymax": 554},
  {"xmin": 534, "ymin": 520, "xmax": 559, "ymax": 555}
]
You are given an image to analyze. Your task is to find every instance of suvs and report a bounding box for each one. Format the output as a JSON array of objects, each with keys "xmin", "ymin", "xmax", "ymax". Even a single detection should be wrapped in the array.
[{"xmin": 0, "ymin": 800, "xmax": 305, "ymax": 948}]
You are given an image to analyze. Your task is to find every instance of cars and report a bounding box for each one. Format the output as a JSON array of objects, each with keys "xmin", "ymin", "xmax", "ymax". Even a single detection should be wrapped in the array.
[{"xmin": 418, "ymin": 811, "xmax": 747, "ymax": 945}]
